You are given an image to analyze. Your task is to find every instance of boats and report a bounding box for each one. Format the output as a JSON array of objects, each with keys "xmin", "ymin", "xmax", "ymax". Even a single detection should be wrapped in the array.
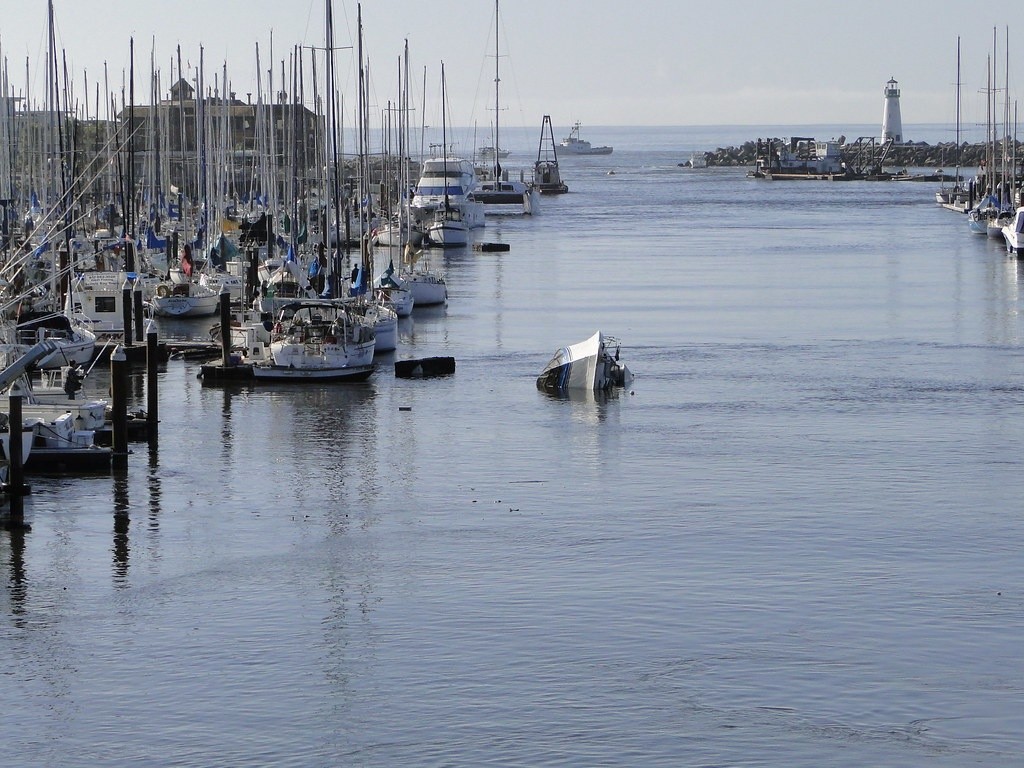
[
  {"xmin": 550, "ymin": 118, "xmax": 614, "ymax": 157},
  {"xmin": 528, "ymin": 316, "xmax": 637, "ymax": 408},
  {"xmin": 0, "ymin": 0, "xmax": 570, "ymax": 510},
  {"xmin": 743, "ymin": 132, "xmax": 915, "ymax": 181},
  {"xmin": 932, "ymin": 20, "xmax": 1024, "ymax": 255}
]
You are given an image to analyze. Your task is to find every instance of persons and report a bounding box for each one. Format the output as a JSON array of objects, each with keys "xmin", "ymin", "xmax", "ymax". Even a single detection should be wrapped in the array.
[
  {"xmin": 351, "ymin": 264, "xmax": 360, "ymax": 284},
  {"xmin": 262, "ymin": 280, "xmax": 268, "ymax": 296},
  {"xmin": 63, "ymin": 360, "xmax": 84, "ymax": 400}
]
